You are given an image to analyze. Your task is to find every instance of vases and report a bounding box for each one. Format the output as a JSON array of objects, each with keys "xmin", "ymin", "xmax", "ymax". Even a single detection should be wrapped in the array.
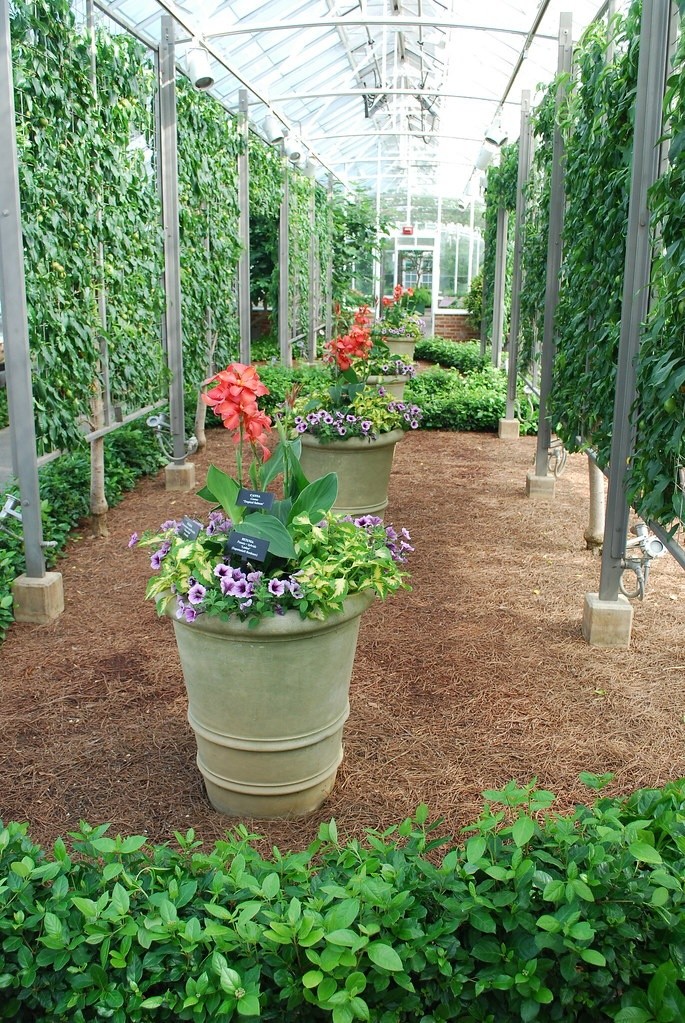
[
  {"xmin": 377, "ymin": 334, "xmax": 419, "ymax": 364},
  {"xmin": 289, "ymin": 431, "xmax": 404, "ymax": 540},
  {"xmin": 151, "ymin": 587, "xmax": 370, "ymax": 820},
  {"xmin": 371, "ymin": 374, "xmax": 411, "ymax": 400}
]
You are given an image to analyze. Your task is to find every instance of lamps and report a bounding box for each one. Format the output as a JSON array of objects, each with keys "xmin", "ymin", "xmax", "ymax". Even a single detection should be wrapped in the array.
[
  {"xmin": 185, "ymin": 38, "xmax": 214, "ymax": 92},
  {"xmin": 485, "ymin": 126, "xmax": 508, "ymax": 146},
  {"xmin": 345, "ymin": 195, "xmax": 356, "ymax": 206},
  {"xmin": 478, "ymin": 148, "xmax": 493, "ymax": 171},
  {"xmin": 454, "ymin": 193, "xmax": 474, "ymax": 212},
  {"xmin": 284, "ymin": 136, "xmax": 302, "ymax": 163},
  {"xmin": 464, "ymin": 166, "xmax": 484, "ymax": 195},
  {"xmin": 304, "ymin": 154, "xmax": 316, "ymax": 179},
  {"xmin": 262, "ymin": 113, "xmax": 283, "ymax": 145}
]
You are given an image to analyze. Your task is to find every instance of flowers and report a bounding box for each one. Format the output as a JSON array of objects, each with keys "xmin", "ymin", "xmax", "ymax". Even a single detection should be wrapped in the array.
[{"xmin": 127, "ymin": 308, "xmax": 422, "ymax": 625}]
